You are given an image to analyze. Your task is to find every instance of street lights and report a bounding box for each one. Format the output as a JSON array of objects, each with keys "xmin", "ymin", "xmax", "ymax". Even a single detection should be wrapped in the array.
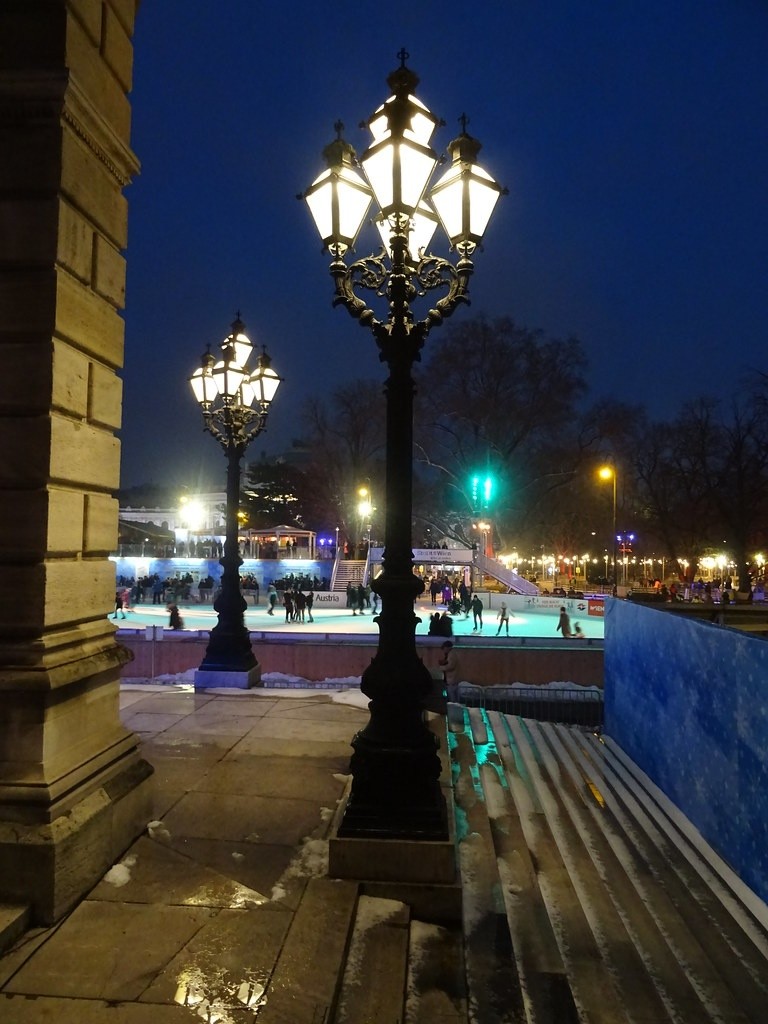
[
  {"xmin": 496, "ymin": 544, "xmax": 766, "ymax": 583},
  {"xmin": 356, "ymin": 475, "xmax": 373, "ymax": 551},
  {"xmin": 305, "ymin": 41, "xmax": 501, "ymax": 837},
  {"xmin": 187, "ymin": 301, "xmax": 279, "ymax": 671},
  {"xmin": 599, "ymin": 465, "xmax": 618, "ymax": 599}
]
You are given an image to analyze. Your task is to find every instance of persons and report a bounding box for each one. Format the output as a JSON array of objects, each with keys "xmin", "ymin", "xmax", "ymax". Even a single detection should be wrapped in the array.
[{"xmin": 113, "ymin": 538, "xmax": 763, "ymax": 638}]
